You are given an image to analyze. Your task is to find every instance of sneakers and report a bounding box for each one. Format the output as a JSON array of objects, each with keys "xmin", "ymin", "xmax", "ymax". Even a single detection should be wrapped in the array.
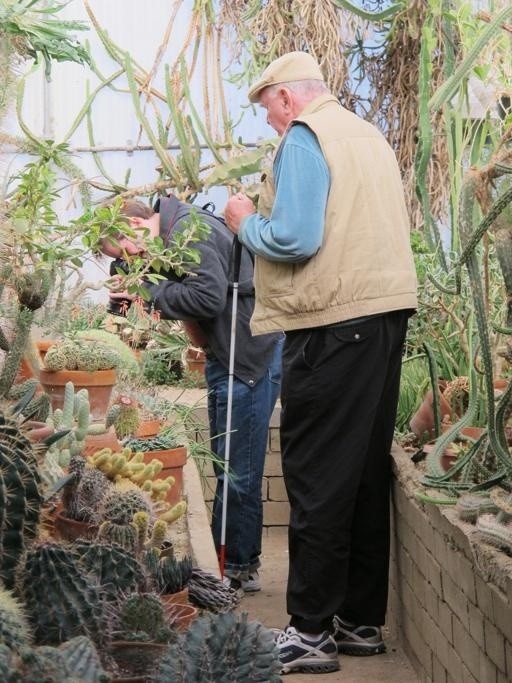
[
  {"xmin": 273, "ymin": 625, "xmax": 340, "ymax": 674},
  {"xmin": 222, "ymin": 568, "xmax": 262, "ymax": 598},
  {"xmin": 333, "ymin": 615, "xmax": 386, "ymax": 656}
]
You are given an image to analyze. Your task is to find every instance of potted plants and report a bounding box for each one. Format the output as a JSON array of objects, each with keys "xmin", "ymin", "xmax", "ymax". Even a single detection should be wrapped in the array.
[
  {"xmin": 410, "ymin": 345, "xmax": 512, "ymax": 475},
  {"xmin": 12, "ymin": 330, "xmax": 207, "ymax": 673}
]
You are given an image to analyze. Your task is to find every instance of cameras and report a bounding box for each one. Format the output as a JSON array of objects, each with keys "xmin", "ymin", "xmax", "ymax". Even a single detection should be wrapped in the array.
[{"xmin": 107, "ymin": 259, "xmax": 140, "ymax": 318}]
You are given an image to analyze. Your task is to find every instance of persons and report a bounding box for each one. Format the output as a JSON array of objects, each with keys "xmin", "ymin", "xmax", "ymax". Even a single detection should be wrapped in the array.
[
  {"xmin": 96, "ymin": 193, "xmax": 286, "ymax": 598},
  {"xmin": 225, "ymin": 51, "xmax": 417, "ymax": 676}
]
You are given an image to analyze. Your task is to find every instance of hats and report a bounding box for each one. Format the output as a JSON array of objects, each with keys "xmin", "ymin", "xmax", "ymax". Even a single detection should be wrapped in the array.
[{"xmin": 247, "ymin": 50, "xmax": 324, "ymax": 102}]
